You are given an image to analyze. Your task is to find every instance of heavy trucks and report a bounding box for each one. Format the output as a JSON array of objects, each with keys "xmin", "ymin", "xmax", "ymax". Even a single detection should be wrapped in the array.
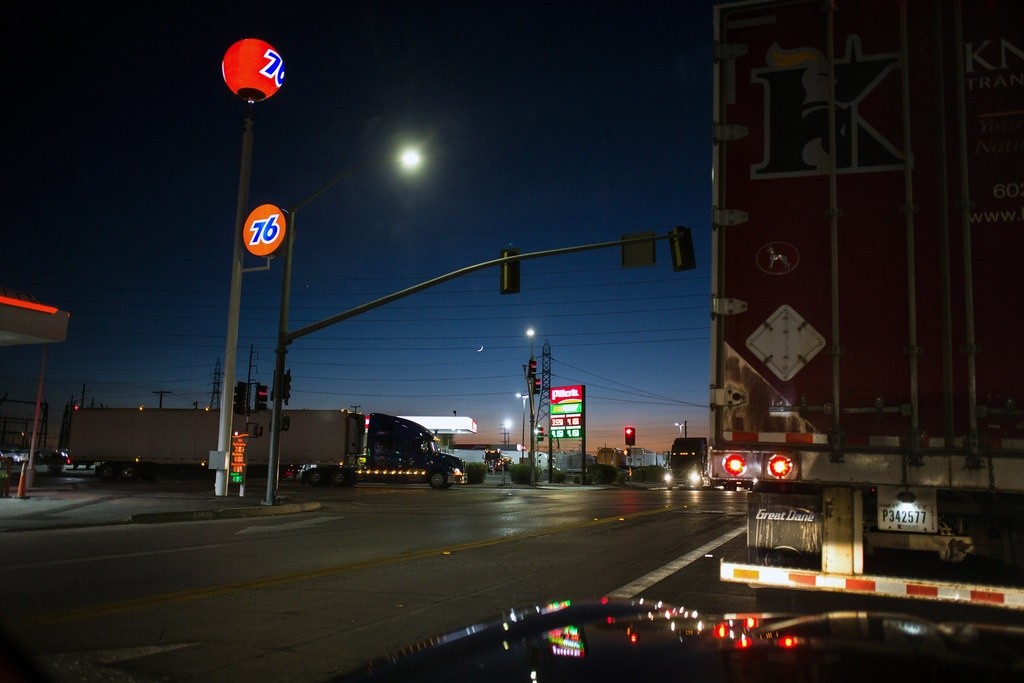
[{"xmin": 68, "ymin": 406, "xmax": 469, "ymax": 491}]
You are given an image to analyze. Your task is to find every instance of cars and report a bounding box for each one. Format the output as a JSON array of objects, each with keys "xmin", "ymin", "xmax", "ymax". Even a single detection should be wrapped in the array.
[
  {"xmin": 310, "ymin": 597, "xmax": 1024, "ymax": 683},
  {"xmin": 0, "ymin": 449, "xmax": 72, "ymax": 466}
]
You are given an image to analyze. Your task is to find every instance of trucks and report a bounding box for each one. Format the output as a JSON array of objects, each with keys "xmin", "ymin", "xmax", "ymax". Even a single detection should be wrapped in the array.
[
  {"xmin": 483, "ymin": 449, "xmax": 513, "ymax": 470},
  {"xmin": 666, "ymin": 435, "xmax": 708, "ymax": 491},
  {"xmin": 700, "ymin": 0, "xmax": 1024, "ymax": 629}
]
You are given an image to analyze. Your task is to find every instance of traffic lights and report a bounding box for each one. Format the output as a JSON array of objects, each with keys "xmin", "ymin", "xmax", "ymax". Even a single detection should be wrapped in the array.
[
  {"xmin": 626, "ymin": 426, "xmax": 636, "ymax": 446},
  {"xmin": 234, "ymin": 381, "xmax": 247, "ymax": 413},
  {"xmin": 255, "ymin": 382, "xmax": 268, "ymax": 411},
  {"xmin": 527, "ymin": 359, "xmax": 536, "ymax": 379},
  {"xmin": 534, "ymin": 377, "xmax": 542, "ymax": 394},
  {"xmin": 536, "ymin": 425, "xmax": 546, "ymax": 442}
]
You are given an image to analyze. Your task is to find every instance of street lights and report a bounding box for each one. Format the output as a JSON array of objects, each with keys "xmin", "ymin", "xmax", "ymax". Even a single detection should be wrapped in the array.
[
  {"xmin": 527, "ymin": 328, "xmax": 536, "ymax": 485},
  {"xmin": 262, "ymin": 150, "xmax": 421, "ymax": 505}
]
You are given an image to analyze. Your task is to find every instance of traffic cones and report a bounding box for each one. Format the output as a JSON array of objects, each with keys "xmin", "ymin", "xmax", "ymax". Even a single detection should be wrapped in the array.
[{"xmin": 11, "ymin": 462, "xmax": 30, "ymax": 499}]
